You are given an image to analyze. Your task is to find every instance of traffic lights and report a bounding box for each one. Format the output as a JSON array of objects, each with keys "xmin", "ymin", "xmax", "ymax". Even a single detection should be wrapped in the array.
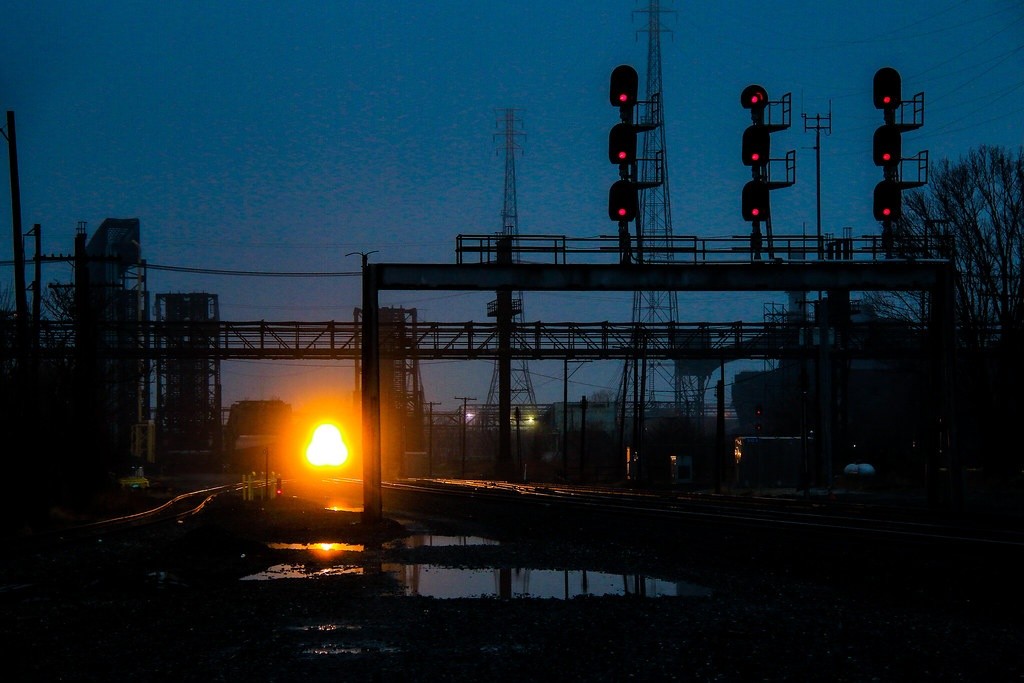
[
  {"xmin": 607, "ymin": 66, "xmax": 641, "ymax": 106},
  {"xmin": 740, "ymin": 123, "xmax": 772, "ymax": 171},
  {"xmin": 608, "ymin": 118, "xmax": 638, "ymax": 167},
  {"xmin": 604, "ymin": 178, "xmax": 642, "ymax": 224},
  {"xmin": 739, "ymin": 82, "xmax": 770, "ymax": 111},
  {"xmin": 871, "ymin": 67, "xmax": 903, "ymax": 112},
  {"xmin": 740, "ymin": 179, "xmax": 772, "ymax": 222},
  {"xmin": 872, "ymin": 180, "xmax": 905, "ymax": 224},
  {"xmin": 871, "ymin": 123, "xmax": 903, "ymax": 169}
]
